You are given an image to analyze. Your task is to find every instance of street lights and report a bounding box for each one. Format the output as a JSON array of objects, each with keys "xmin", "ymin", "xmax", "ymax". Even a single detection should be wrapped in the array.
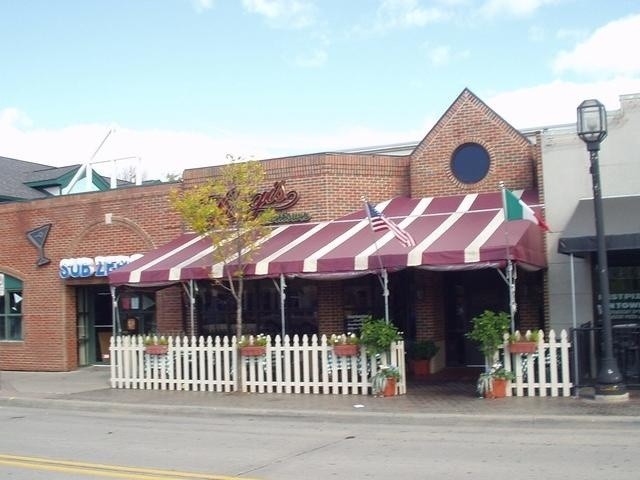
[{"xmin": 575, "ymin": 98, "xmax": 630, "ymax": 403}]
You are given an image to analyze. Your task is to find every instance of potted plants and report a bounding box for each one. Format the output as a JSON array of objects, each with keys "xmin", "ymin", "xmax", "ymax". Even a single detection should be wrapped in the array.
[
  {"xmin": 141, "ymin": 335, "xmax": 167, "ymax": 355},
  {"xmin": 478, "ymin": 369, "xmax": 515, "ymax": 399},
  {"xmin": 410, "ymin": 341, "xmax": 440, "ymax": 375},
  {"xmin": 374, "ymin": 366, "xmax": 400, "ymax": 396}
]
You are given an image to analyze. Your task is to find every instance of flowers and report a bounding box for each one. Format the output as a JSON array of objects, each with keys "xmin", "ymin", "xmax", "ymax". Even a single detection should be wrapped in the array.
[
  {"xmin": 327, "ymin": 331, "xmax": 356, "ymax": 345},
  {"xmin": 238, "ymin": 333, "xmax": 267, "ymax": 346}
]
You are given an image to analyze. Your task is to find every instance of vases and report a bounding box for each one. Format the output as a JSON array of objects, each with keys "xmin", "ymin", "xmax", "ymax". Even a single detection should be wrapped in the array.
[
  {"xmin": 335, "ymin": 345, "xmax": 357, "ymax": 356},
  {"xmin": 240, "ymin": 346, "xmax": 265, "ymax": 356}
]
